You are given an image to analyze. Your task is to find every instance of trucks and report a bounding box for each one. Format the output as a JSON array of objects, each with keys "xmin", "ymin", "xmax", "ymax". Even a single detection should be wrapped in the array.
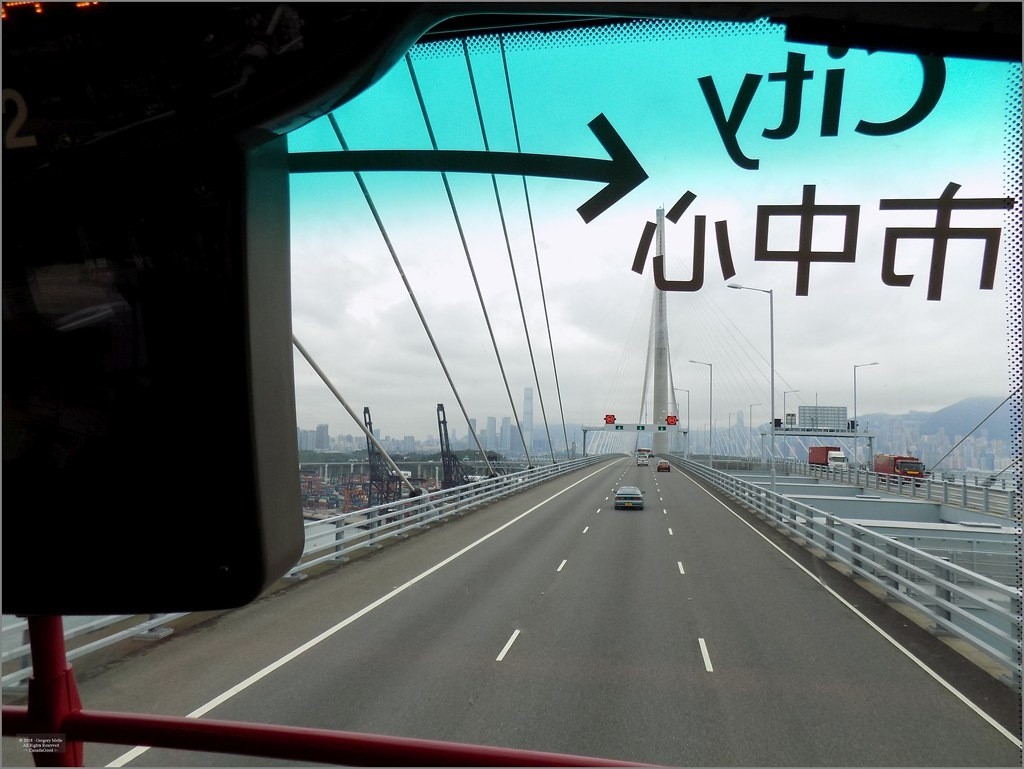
[
  {"xmin": 874, "ymin": 453, "xmax": 926, "ymax": 487},
  {"xmin": 807, "ymin": 445, "xmax": 850, "ymax": 474}
]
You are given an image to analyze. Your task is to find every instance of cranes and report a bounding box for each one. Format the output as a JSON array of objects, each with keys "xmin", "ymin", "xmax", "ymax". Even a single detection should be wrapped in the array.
[
  {"xmin": 435, "ymin": 401, "xmax": 481, "ymax": 493},
  {"xmin": 361, "ymin": 405, "xmax": 403, "ymax": 517}
]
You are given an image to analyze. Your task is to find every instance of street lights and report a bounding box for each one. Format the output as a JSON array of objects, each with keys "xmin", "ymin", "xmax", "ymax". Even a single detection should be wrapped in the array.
[
  {"xmin": 674, "ymin": 388, "xmax": 691, "ymax": 460},
  {"xmin": 727, "ymin": 412, "xmax": 740, "ymax": 460},
  {"xmin": 749, "ymin": 403, "xmax": 765, "ymax": 462},
  {"xmin": 726, "ymin": 283, "xmax": 778, "ymax": 518},
  {"xmin": 853, "ymin": 361, "xmax": 880, "ymax": 470},
  {"xmin": 783, "ymin": 389, "xmax": 802, "ymax": 462},
  {"xmin": 688, "ymin": 360, "xmax": 713, "ymax": 468}
]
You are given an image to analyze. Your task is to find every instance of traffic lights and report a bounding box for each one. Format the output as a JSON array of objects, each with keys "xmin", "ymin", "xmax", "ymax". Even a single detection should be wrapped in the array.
[
  {"xmin": 637, "ymin": 425, "xmax": 645, "ymax": 430},
  {"xmin": 657, "ymin": 426, "xmax": 666, "ymax": 431},
  {"xmin": 616, "ymin": 425, "xmax": 623, "ymax": 430}
]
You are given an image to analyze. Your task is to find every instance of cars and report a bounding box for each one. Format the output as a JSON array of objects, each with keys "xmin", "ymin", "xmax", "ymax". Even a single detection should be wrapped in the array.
[
  {"xmin": 635, "ymin": 448, "xmax": 654, "ymax": 466},
  {"xmin": 656, "ymin": 460, "xmax": 671, "ymax": 472},
  {"xmin": 613, "ymin": 486, "xmax": 645, "ymax": 510}
]
